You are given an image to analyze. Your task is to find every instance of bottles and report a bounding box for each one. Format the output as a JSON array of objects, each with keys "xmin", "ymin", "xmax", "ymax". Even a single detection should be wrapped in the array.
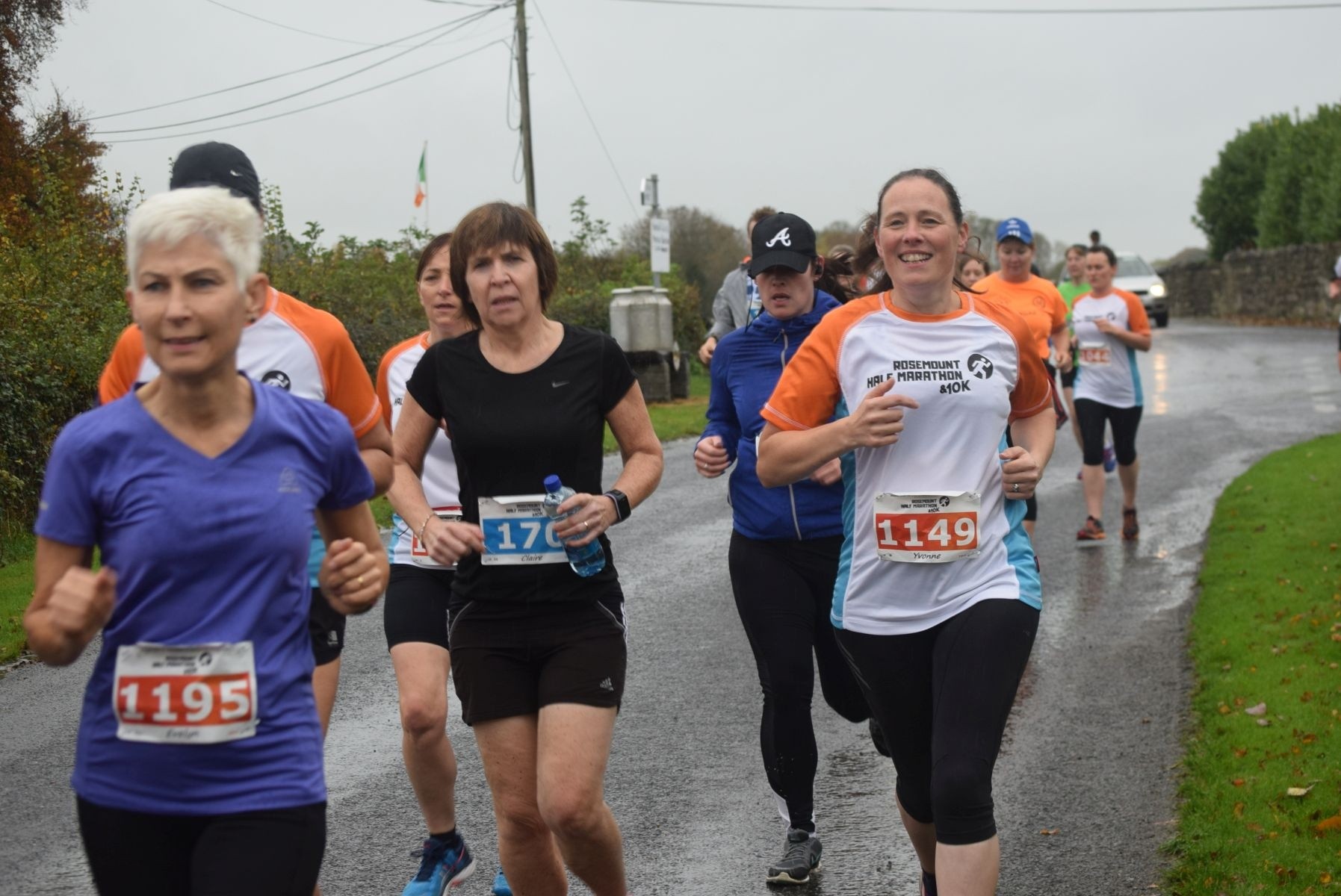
[{"xmin": 542, "ymin": 474, "xmax": 606, "ymax": 577}]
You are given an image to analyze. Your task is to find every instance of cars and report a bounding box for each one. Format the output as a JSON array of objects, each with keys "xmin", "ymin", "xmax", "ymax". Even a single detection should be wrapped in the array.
[{"xmin": 1057, "ymin": 251, "xmax": 1174, "ymax": 326}]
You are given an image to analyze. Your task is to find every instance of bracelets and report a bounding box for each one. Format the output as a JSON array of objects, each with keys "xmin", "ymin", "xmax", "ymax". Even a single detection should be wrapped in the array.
[{"xmin": 419, "ymin": 512, "xmax": 436, "ymax": 540}]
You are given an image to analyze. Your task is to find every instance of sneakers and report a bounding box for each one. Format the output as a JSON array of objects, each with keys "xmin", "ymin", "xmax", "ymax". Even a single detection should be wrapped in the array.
[
  {"xmin": 869, "ymin": 717, "xmax": 891, "ymax": 756},
  {"xmin": 493, "ymin": 864, "xmax": 512, "ymax": 896},
  {"xmin": 1077, "ymin": 516, "xmax": 1106, "ymax": 547},
  {"xmin": 402, "ymin": 832, "xmax": 476, "ymax": 896},
  {"xmin": 1122, "ymin": 507, "xmax": 1138, "ymax": 540},
  {"xmin": 1105, "ymin": 446, "xmax": 1117, "ymax": 473},
  {"xmin": 766, "ymin": 830, "xmax": 822, "ymax": 883}
]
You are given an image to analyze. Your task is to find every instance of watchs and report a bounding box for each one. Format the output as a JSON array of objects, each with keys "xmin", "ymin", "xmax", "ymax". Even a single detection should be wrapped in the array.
[{"xmin": 603, "ymin": 489, "xmax": 630, "ymax": 526}]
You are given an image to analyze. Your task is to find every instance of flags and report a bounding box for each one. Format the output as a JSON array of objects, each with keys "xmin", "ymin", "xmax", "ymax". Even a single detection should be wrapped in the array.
[{"xmin": 411, "ymin": 153, "xmax": 427, "ymax": 207}]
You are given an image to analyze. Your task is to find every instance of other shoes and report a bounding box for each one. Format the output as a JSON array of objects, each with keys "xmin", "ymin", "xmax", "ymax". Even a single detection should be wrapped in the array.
[{"xmin": 920, "ymin": 872, "xmax": 938, "ymax": 896}]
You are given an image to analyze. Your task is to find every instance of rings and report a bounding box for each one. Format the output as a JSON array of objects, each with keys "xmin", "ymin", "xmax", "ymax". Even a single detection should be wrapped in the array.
[
  {"xmin": 1014, "ymin": 484, "xmax": 1018, "ymax": 492},
  {"xmin": 585, "ymin": 522, "xmax": 588, "ymax": 530},
  {"xmin": 705, "ymin": 463, "xmax": 708, "ymax": 469},
  {"xmin": 358, "ymin": 577, "xmax": 364, "ymax": 587}
]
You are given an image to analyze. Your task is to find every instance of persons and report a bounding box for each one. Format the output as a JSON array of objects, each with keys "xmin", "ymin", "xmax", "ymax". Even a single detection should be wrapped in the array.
[
  {"xmin": 756, "ymin": 168, "xmax": 1055, "ymax": 896},
  {"xmin": 96, "ymin": 142, "xmax": 395, "ymax": 746},
  {"xmin": 972, "ymin": 218, "xmax": 1070, "ymax": 547},
  {"xmin": 827, "ymin": 245, "xmax": 884, "ymax": 299},
  {"xmin": 26, "ymin": 185, "xmax": 389, "ymax": 895},
  {"xmin": 693, "ymin": 211, "xmax": 891, "ymax": 886},
  {"xmin": 1076, "ymin": 231, "xmax": 1150, "ymax": 541},
  {"xmin": 1056, "ymin": 244, "xmax": 1118, "ymax": 481},
  {"xmin": 697, "ymin": 207, "xmax": 778, "ymax": 366},
  {"xmin": 961, "ymin": 257, "xmax": 987, "ymax": 291},
  {"xmin": 378, "ymin": 233, "xmax": 516, "ymax": 895},
  {"xmin": 387, "ymin": 203, "xmax": 662, "ymax": 895}
]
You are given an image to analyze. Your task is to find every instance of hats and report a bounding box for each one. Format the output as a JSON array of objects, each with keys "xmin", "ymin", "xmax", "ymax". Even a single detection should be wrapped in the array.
[
  {"xmin": 998, "ymin": 218, "xmax": 1032, "ymax": 245},
  {"xmin": 751, "ymin": 212, "xmax": 816, "ymax": 278},
  {"xmin": 170, "ymin": 142, "xmax": 259, "ymax": 208}
]
